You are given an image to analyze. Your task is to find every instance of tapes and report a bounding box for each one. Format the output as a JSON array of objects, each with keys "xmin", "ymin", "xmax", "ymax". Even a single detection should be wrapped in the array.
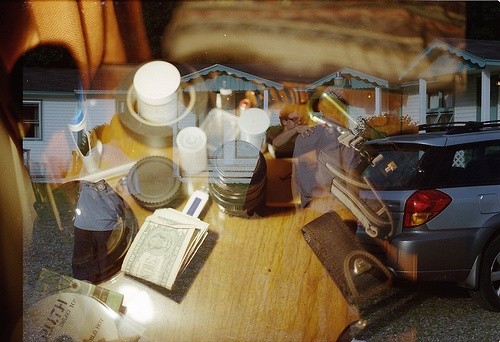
[{"xmin": 116, "ymin": 58, "xmax": 210, "ymax": 148}]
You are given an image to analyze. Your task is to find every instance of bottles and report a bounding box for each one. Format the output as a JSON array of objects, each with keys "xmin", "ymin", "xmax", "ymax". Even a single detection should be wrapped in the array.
[{"xmin": 133, "ymin": 60, "xmax": 181, "ymax": 121}]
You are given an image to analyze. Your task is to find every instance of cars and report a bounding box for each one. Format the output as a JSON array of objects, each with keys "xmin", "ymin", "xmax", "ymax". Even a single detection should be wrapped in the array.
[{"xmin": 356, "ymin": 120, "xmax": 500, "ymax": 311}]
